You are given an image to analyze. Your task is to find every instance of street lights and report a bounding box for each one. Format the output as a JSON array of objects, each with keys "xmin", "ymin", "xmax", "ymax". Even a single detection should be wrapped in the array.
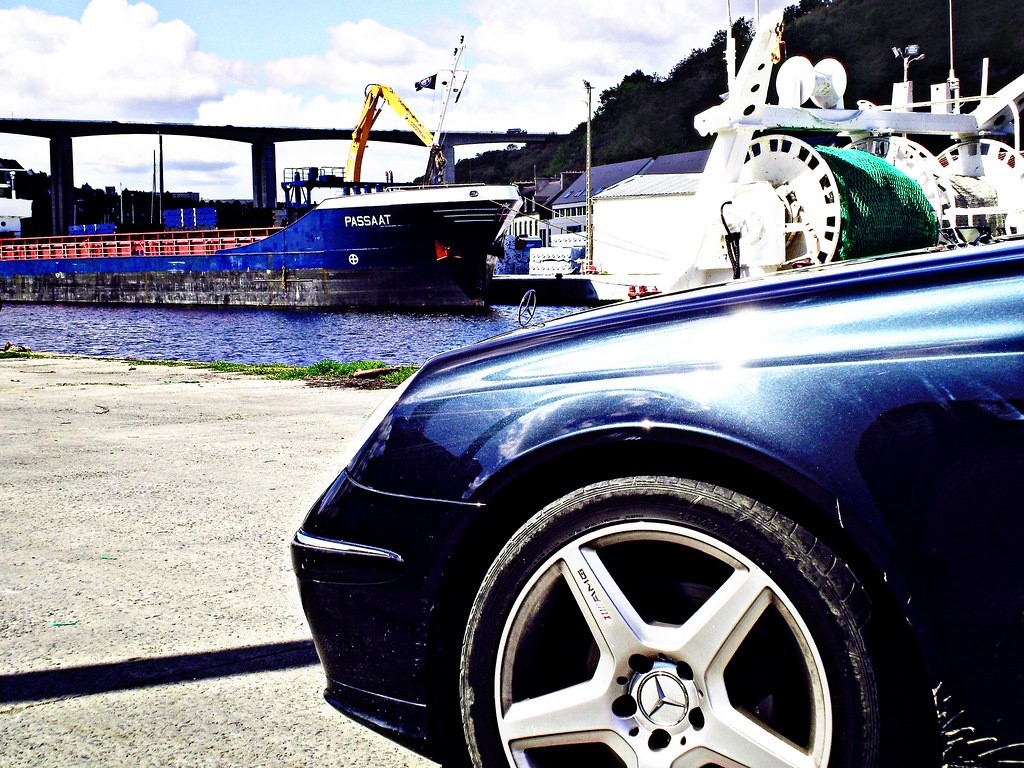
[{"xmin": 582, "ymin": 79, "xmax": 593, "ymax": 272}]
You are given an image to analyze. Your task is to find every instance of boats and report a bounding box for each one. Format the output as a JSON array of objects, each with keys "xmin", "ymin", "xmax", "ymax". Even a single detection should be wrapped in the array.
[{"xmin": 0, "ymin": 33, "xmax": 525, "ymax": 317}]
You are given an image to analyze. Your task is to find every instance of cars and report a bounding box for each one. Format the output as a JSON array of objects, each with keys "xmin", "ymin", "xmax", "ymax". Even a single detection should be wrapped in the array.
[{"xmin": 286, "ymin": 230, "xmax": 1024, "ymax": 768}]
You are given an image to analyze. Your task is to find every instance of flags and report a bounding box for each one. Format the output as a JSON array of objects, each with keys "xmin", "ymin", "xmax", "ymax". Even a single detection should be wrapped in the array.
[{"xmin": 414, "ymin": 75, "xmax": 437, "ymax": 91}]
